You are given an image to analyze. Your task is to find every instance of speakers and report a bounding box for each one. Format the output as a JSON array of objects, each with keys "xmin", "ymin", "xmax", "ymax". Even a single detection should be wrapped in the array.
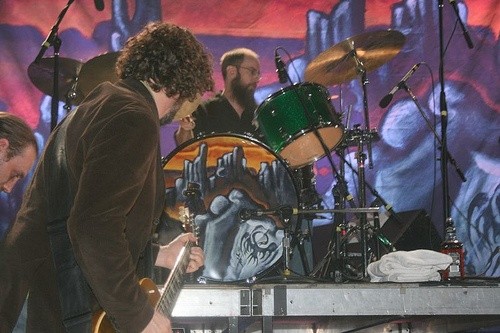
[{"xmin": 336, "ymin": 208, "xmax": 442, "ymax": 282}]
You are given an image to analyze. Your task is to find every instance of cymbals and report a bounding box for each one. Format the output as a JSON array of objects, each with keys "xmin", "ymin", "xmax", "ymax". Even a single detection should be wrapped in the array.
[
  {"xmin": 302, "ymin": 29, "xmax": 406, "ymax": 88},
  {"xmin": 78, "ymin": 51, "xmax": 201, "ymax": 124},
  {"xmin": 27, "ymin": 56, "xmax": 84, "ymax": 107}
]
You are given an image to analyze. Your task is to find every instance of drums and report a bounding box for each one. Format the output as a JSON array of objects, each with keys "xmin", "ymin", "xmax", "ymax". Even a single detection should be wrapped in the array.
[
  {"xmin": 158, "ymin": 133, "xmax": 303, "ymax": 284},
  {"xmin": 256, "ymin": 81, "xmax": 345, "ymax": 169},
  {"xmin": 289, "ymin": 165, "xmax": 323, "ymax": 209}
]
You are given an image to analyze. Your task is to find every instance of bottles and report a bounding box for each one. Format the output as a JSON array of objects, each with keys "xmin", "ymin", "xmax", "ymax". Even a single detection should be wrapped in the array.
[{"xmin": 441, "ymin": 217, "xmax": 466, "ymax": 283}]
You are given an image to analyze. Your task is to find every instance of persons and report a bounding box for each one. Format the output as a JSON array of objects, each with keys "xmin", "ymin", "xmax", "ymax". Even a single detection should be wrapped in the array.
[
  {"xmin": 0, "ymin": 22, "xmax": 215, "ymax": 333},
  {"xmin": 174, "ymin": 47, "xmax": 313, "ymax": 279},
  {"xmin": 0, "ymin": 112, "xmax": 39, "ymax": 193}
]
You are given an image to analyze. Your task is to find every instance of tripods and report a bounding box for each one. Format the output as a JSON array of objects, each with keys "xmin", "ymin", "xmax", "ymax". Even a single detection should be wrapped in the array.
[{"xmin": 309, "ymin": 139, "xmax": 399, "ymax": 281}]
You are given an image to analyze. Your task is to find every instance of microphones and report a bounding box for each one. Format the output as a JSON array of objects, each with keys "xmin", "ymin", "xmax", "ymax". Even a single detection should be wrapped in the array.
[
  {"xmin": 379, "ymin": 63, "xmax": 421, "ymax": 108},
  {"xmin": 94, "ymin": 0, "xmax": 104, "ymax": 10},
  {"xmin": 238, "ymin": 207, "xmax": 253, "ymax": 220},
  {"xmin": 275, "ymin": 52, "xmax": 288, "ymax": 83}
]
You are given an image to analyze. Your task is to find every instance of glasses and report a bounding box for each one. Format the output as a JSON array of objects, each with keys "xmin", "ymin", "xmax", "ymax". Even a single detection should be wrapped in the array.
[{"xmin": 232, "ymin": 65, "xmax": 262, "ymax": 81}]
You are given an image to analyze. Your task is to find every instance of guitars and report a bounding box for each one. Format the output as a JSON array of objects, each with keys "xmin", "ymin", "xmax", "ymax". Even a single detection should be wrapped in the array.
[{"xmin": 87, "ymin": 205, "xmax": 201, "ymax": 332}]
